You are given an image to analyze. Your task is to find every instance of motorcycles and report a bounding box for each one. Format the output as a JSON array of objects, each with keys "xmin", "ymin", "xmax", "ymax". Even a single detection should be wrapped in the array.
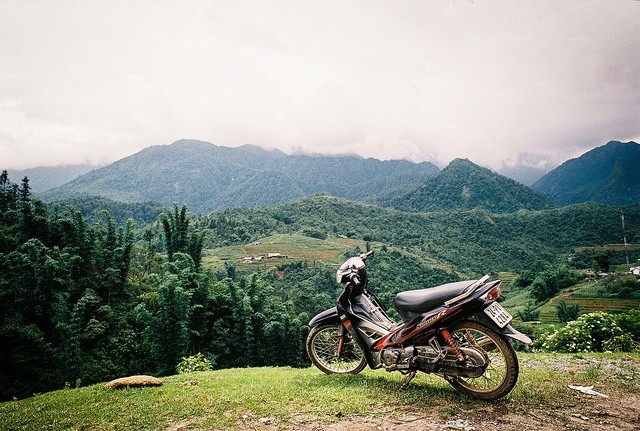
[{"xmin": 305, "ymin": 250, "xmax": 535, "ymax": 402}]
